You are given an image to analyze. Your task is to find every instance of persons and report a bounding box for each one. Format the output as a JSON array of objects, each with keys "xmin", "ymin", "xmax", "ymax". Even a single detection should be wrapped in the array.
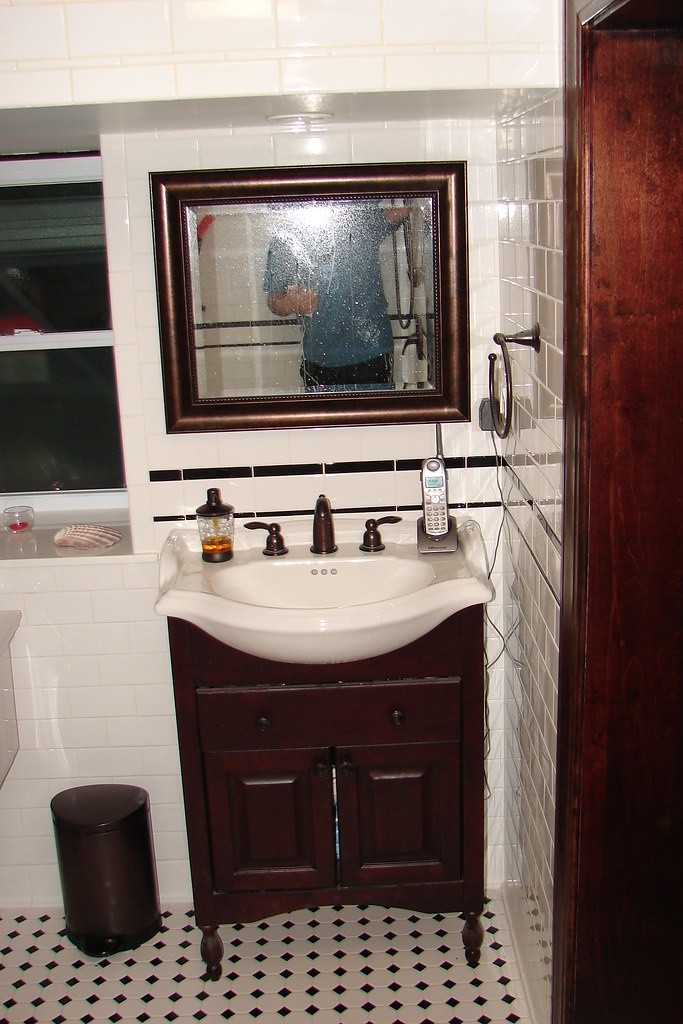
[{"xmin": 263, "ymin": 199, "xmax": 411, "ymax": 385}]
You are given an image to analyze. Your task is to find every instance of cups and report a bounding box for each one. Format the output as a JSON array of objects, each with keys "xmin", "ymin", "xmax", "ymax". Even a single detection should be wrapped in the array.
[{"xmin": 3, "ymin": 505, "xmax": 35, "ymax": 534}]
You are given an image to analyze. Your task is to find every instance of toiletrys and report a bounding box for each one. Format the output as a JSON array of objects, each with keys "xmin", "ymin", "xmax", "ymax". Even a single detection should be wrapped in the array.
[{"xmin": 195, "ymin": 487, "xmax": 236, "ymax": 563}]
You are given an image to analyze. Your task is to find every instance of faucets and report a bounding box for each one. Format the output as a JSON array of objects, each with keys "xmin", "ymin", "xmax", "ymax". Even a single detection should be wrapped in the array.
[{"xmin": 310, "ymin": 493, "xmax": 338, "ymax": 555}]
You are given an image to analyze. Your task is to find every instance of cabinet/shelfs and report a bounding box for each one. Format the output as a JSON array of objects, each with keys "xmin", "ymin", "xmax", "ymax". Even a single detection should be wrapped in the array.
[{"xmin": 167, "ymin": 602, "xmax": 484, "ymax": 977}]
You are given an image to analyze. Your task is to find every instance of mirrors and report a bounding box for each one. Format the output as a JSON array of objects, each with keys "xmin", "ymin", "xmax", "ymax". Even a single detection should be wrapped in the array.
[{"xmin": 149, "ymin": 160, "xmax": 471, "ymax": 434}]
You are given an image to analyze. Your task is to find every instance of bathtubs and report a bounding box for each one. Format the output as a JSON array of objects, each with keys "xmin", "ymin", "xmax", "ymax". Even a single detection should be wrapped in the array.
[{"xmin": 200, "ymin": 348, "xmax": 406, "ymax": 393}]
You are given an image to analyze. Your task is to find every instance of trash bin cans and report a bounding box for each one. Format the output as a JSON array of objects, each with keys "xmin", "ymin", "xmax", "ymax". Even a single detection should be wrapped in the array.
[{"xmin": 51, "ymin": 783, "xmax": 162, "ymax": 957}]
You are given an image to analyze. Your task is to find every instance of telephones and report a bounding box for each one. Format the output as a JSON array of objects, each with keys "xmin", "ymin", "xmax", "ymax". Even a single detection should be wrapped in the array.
[{"xmin": 417, "ymin": 423, "xmax": 457, "ymax": 554}]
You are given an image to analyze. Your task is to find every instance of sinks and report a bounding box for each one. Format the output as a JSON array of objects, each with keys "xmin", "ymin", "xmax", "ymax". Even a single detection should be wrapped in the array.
[{"xmin": 154, "ymin": 560, "xmax": 493, "ymax": 665}]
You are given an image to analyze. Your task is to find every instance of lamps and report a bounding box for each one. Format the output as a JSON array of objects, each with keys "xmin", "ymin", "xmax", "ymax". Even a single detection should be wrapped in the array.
[{"xmin": 196, "ymin": 487, "xmax": 234, "ymax": 563}]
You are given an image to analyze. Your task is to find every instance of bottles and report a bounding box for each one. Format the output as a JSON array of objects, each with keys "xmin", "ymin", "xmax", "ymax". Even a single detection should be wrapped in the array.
[{"xmin": 195, "ymin": 487, "xmax": 236, "ymax": 563}]
